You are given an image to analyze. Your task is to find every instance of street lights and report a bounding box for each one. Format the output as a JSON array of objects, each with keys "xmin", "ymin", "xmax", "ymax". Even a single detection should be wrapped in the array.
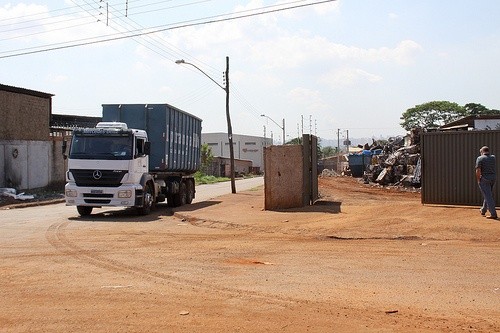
[
  {"xmin": 260, "ymin": 114, "xmax": 285, "ymax": 144},
  {"xmin": 175, "ymin": 56, "xmax": 237, "ymax": 194}
]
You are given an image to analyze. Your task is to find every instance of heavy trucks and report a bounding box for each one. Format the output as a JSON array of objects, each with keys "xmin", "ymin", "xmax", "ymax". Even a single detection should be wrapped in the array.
[{"xmin": 64, "ymin": 104, "xmax": 203, "ymax": 218}]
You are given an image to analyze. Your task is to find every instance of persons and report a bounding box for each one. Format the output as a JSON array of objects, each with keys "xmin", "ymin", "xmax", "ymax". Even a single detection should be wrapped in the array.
[{"xmin": 475, "ymin": 146, "xmax": 498, "ymax": 219}]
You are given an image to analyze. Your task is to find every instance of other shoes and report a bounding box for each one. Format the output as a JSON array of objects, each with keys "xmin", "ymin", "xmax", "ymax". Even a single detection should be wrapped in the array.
[
  {"xmin": 479, "ymin": 208, "xmax": 485, "ymax": 215},
  {"xmin": 486, "ymin": 215, "xmax": 497, "ymax": 218}
]
